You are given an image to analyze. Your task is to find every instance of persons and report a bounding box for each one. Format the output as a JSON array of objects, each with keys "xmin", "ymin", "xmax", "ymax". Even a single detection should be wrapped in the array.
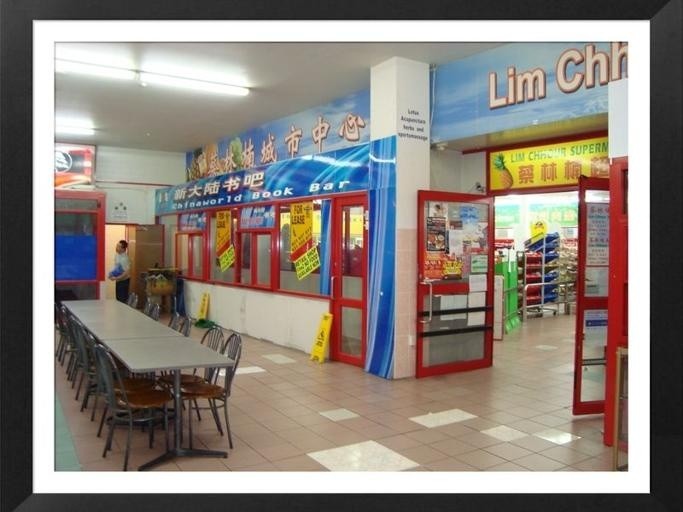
[{"xmin": 110, "ymin": 240, "xmax": 132, "ymax": 305}]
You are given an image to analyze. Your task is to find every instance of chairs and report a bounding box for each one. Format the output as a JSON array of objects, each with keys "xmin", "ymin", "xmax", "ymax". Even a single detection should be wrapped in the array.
[{"xmin": 53, "ymin": 268, "xmax": 245, "ymax": 471}]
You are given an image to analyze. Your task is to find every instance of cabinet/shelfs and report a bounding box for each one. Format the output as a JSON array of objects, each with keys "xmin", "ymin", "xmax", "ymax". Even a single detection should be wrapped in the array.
[{"xmin": 494, "ymin": 229, "xmax": 580, "ymax": 320}]
